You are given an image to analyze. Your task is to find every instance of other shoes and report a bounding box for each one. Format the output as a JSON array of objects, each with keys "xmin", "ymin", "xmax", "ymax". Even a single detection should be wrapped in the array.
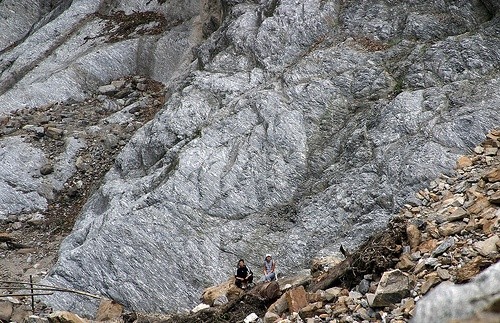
[
  {"xmin": 242, "ymin": 285, "xmax": 247, "ymax": 288},
  {"xmin": 265, "ymin": 279, "xmax": 270, "ymax": 283},
  {"xmin": 248, "ymin": 283, "xmax": 254, "ymax": 287}
]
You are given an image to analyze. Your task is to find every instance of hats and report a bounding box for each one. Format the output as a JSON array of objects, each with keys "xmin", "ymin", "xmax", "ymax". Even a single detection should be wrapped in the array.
[{"xmin": 266, "ymin": 254, "xmax": 271, "ymax": 257}]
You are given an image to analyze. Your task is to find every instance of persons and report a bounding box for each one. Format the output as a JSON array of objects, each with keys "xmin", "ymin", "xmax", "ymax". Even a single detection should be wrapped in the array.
[
  {"xmin": 234, "ymin": 259, "xmax": 255, "ymax": 289},
  {"xmin": 258, "ymin": 253, "xmax": 276, "ymax": 283}
]
